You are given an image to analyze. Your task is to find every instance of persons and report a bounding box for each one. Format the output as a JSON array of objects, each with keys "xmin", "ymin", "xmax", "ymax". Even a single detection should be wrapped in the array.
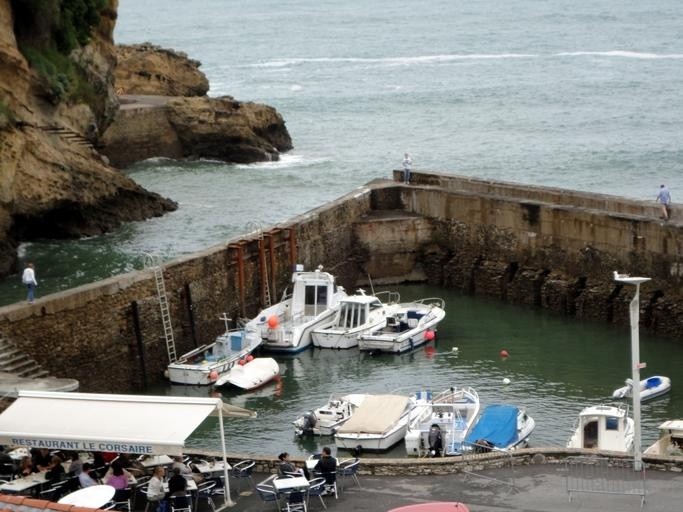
[
  {"xmin": 0, "ymin": 445, "xmax": 192, "ymax": 512},
  {"xmin": 278, "ymin": 452, "xmax": 310, "ymax": 481},
  {"xmin": 21, "ymin": 263, "xmax": 37, "ymax": 301},
  {"xmin": 656, "ymin": 184, "xmax": 671, "ymax": 220},
  {"xmin": 312, "ymin": 447, "xmax": 336, "ymax": 496},
  {"xmin": 402, "ymin": 153, "xmax": 412, "ymax": 185}
]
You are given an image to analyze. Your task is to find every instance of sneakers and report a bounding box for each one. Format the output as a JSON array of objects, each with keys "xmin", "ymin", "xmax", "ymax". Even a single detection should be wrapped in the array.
[
  {"xmin": 330, "ymin": 488, "xmax": 335, "ymax": 496},
  {"xmin": 320, "ymin": 490, "xmax": 328, "ymax": 497}
]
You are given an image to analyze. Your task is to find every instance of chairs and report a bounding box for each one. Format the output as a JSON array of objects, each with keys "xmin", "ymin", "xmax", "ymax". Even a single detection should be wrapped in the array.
[
  {"xmin": 1, "ymin": 446, "xmax": 231, "ymax": 512},
  {"xmin": 257, "ymin": 456, "xmax": 361, "ymax": 512},
  {"xmin": 231, "ymin": 458, "xmax": 257, "ymax": 496}
]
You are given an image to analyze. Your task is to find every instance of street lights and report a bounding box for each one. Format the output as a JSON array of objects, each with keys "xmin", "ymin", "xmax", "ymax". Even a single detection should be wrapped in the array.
[{"xmin": 607, "ymin": 268, "xmax": 657, "ymax": 473}]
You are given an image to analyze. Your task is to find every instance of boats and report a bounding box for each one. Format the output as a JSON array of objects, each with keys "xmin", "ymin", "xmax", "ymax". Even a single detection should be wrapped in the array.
[
  {"xmin": 214, "ymin": 375, "xmax": 281, "ymax": 402},
  {"xmin": 243, "ymin": 267, "xmax": 348, "ymax": 356},
  {"xmin": 213, "ymin": 355, "xmax": 282, "ymax": 393},
  {"xmin": 402, "ymin": 386, "xmax": 481, "ymax": 456},
  {"xmin": 615, "ymin": 372, "xmax": 671, "ymax": 403},
  {"xmin": 561, "ymin": 399, "xmax": 638, "ymax": 456},
  {"xmin": 640, "ymin": 419, "xmax": 683, "ymax": 460},
  {"xmin": 163, "ymin": 313, "xmax": 264, "ymax": 386},
  {"xmin": 460, "ymin": 404, "xmax": 535, "ymax": 457},
  {"xmin": 295, "ymin": 385, "xmax": 433, "ymax": 454},
  {"xmin": 308, "ymin": 293, "xmax": 404, "ymax": 358},
  {"xmin": 354, "ymin": 294, "xmax": 446, "ymax": 358}
]
